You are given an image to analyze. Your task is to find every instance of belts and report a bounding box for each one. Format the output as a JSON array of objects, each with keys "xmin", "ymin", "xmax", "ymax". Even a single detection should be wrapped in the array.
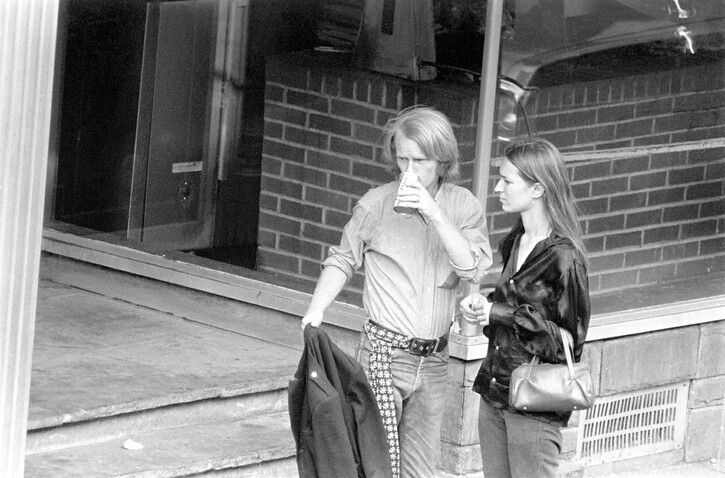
[{"xmin": 363, "ymin": 319, "xmax": 453, "ymax": 357}]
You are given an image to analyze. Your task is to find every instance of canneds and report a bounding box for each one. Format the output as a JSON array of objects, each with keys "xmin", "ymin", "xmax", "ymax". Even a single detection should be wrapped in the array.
[{"xmin": 394, "ymin": 172, "xmax": 421, "ymax": 213}]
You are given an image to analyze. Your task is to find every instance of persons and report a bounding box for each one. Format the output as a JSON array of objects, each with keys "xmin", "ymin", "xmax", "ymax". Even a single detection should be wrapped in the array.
[
  {"xmin": 300, "ymin": 108, "xmax": 494, "ymax": 478},
  {"xmin": 458, "ymin": 136, "xmax": 592, "ymax": 478}
]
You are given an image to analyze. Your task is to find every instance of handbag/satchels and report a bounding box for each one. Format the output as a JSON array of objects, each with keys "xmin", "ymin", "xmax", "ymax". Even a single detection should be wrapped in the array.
[
  {"xmin": 509, "ymin": 327, "xmax": 595, "ymax": 412},
  {"xmin": 349, "ymin": 0, "xmax": 438, "ymax": 81}
]
